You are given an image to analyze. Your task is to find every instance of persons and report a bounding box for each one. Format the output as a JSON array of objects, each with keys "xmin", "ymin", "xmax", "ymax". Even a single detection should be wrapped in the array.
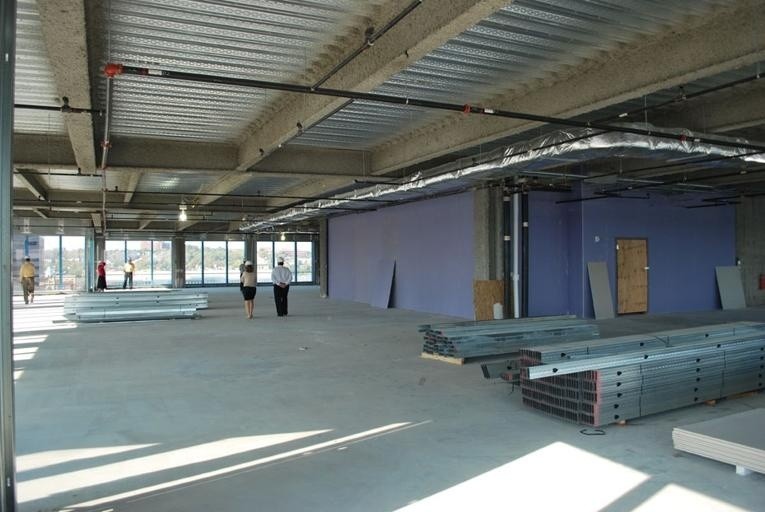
[
  {"xmin": 94, "ymin": 260, "xmax": 107, "ymax": 292},
  {"xmin": 122, "ymin": 258, "xmax": 135, "ymax": 289},
  {"xmin": 240, "ymin": 260, "xmax": 257, "ymax": 319},
  {"xmin": 269, "ymin": 256, "xmax": 292, "ymax": 318},
  {"xmin": 239, "ymin": 260, "xmax": 247, "ymax": 290},
  {"xmin": 19, "ymin": 256, "xmax": 35, "ymax": 305}
]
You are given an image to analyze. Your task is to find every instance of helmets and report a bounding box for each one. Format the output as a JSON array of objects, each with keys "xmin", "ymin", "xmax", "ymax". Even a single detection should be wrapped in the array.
[
  {"xmin": 277, "ymin": 257, "xmax": 283, "ymax": 262},
  {"xmin": 243, "ymin": 258, "xmax": 253, "ymax": 266}
]
